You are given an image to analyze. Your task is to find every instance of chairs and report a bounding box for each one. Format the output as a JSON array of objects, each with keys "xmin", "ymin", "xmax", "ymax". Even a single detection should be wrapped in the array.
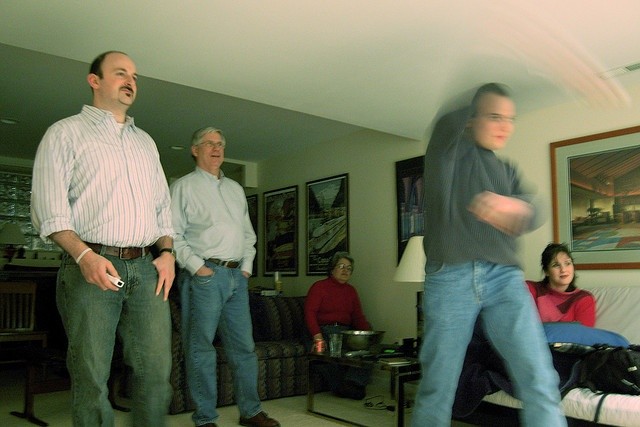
[{"xmin": 0, "ymin": 283, "xmax": 45, "ymax": 425}]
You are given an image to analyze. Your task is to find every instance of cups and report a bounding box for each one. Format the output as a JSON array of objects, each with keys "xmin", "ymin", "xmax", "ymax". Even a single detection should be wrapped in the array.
[{"xmin": 327, "ymin": 332, "xmax": 344, "ymax": 357}]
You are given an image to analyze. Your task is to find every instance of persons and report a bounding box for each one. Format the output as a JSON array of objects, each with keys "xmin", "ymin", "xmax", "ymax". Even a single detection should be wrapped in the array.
[
  {"xmin": 453, "ymin": 244, "xmax": 596, "ymax": 417},
  {"xmin": 409, "ymin": 80, "xmax": 572, "ymax": 427},
  {"xmin": 29, "ymin": 51, "xmax": 178, "ymax": 425},
  {"xmin": 164, "ymin": 126, "xmax": 282, "ymax": 425},
  {"xmin": 302, "ymin": 251, "xmax": 375, "ymax": 400}
]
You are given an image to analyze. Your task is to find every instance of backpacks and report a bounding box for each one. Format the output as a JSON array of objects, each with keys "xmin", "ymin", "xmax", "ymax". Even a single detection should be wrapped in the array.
[{"xmin": 583, "ymin": 343, "xmax": 640, "ymax": 425}]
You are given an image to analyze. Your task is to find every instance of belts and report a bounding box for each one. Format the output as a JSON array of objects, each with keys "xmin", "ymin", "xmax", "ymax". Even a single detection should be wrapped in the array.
[
  {"xmin": 205, "ymin": 258, "xmax": 241, "ymax": 269},
  {"xmin": 84, "ymin": 242, "xmax": 152, "ymax": 259}
]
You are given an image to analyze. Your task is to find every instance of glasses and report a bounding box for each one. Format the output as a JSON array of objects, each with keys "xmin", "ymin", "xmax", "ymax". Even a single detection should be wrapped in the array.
[
  {"xmin": 333, "ymin": 264, "xmax": 353, "ymax": 270},
  {"xmin": 196, "ymin": 141, "xmax": 225, "ymax": 148}
]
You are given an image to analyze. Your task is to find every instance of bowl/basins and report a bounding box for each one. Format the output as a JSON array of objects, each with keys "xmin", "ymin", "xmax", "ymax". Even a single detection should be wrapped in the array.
[{"xmin": 345, "ymin": 329, "xmax": 386, "ymax": 348}]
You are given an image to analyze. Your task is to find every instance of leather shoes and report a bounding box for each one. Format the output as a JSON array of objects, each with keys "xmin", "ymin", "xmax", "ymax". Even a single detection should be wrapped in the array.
[{"xmin": 240, "ymin": 411, "xmax": 281, "ymax": 427}]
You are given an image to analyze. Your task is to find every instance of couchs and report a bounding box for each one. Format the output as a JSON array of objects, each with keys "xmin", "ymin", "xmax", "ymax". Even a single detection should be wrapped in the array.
[
  {"xmin": 154, "ymin": 287, "xmax": 369, "ymax": 415},
  {"xmin": 479, "ymin": 285, "xmax": 636, "ymax": 426}
]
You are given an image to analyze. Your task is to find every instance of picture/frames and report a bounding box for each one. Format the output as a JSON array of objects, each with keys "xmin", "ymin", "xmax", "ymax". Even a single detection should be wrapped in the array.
[
  {"xmin": 245, "ymin": 195, "xmax": 258, "ymax": 274},
  {"xmin": 395, "ymin": 156, "xmax": 427, "ymax": 267},
  {"xmin": 549, "ymin": 125, "xmax": 637, "ymax": 272},
  {"xmin": 262, "ymin": 186, "xmax": 298, "ymax": 277},
  {"xmin": 306, "ymin": 175, "xmax": 351, "ymax": 275}
]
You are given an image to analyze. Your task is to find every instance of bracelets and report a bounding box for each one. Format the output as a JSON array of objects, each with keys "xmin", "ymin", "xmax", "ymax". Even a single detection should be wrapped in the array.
[{"xmin": 75, "ymin": 247, "xmax": 92, "ymax": 263}]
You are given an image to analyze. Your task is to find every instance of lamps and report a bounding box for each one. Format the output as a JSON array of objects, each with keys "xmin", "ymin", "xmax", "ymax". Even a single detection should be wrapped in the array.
[
  {"xmin": 1, "ymin": 222, "xmax": 24, "ymax": 267},
  {"xmin": 392, "ymin": 234, "xmax": 428, "ymax": 284}
]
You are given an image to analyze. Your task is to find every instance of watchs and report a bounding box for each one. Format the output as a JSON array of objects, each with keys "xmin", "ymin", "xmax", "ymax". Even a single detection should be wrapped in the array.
[{"xmin": 159, "ymin": 248, "xmax": 176, "ymax": 258}]
